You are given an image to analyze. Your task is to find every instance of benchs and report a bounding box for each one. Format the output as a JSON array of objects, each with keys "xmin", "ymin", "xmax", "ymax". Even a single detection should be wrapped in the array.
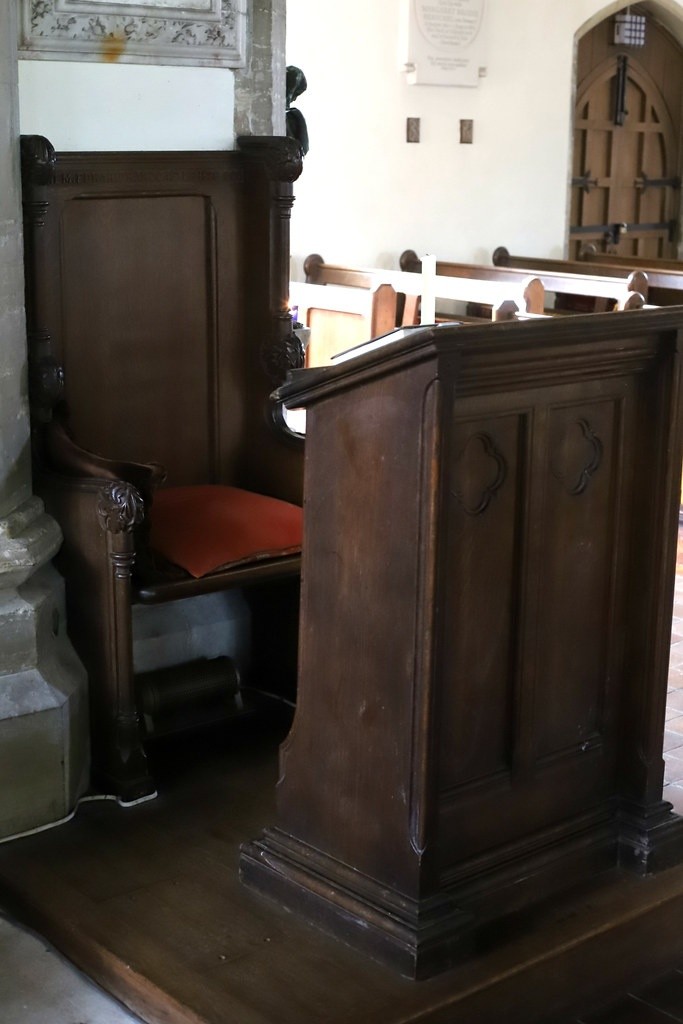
[{"xmin": 290, "ymin": 238, "xmax": 683, "ymax": 370}]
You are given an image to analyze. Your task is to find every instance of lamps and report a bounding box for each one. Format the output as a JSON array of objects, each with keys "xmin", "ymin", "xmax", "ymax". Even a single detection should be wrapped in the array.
[{"xmin": 614, "ymin": 6, "xmax": 647, "ymax": 46}]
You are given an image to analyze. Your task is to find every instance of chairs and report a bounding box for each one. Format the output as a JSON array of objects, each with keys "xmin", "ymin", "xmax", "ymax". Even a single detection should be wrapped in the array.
[{"xmin": 20, "ymin": 134, "xmax": 308, "ymax": 803}]
